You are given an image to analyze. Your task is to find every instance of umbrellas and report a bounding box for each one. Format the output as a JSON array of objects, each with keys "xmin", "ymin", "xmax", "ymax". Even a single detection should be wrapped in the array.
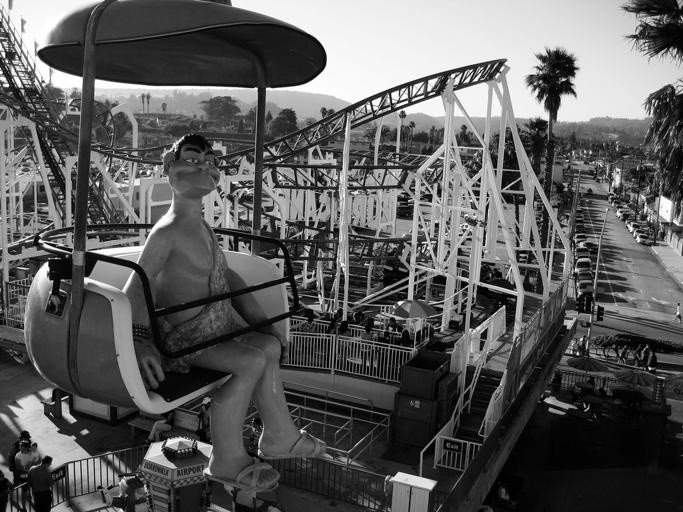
[
  {"xmin": 390, "ymin": 299, "xmax": 440, "ymax": 320},
  {"xmin": 613, "ymin": 368, "xmax": 657, "ymax": 393},
  {"xmin": 566, "ymin": 355, "xmax": 609, "ymax": 384}
]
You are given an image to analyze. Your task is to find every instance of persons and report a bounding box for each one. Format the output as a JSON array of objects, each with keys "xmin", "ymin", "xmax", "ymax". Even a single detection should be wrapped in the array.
[
  {"xmin": 196, "ymin": 396, "xmax": 212, "ymax": 445},
  {"xmin": 616, "ymin": 344, "xmax": 627, "ymax": 365},
  {"xmin": 641, "ymin": 342, "xmax": 650, "ymax": 371},
  {"xmin": 671, "ymin": 302, "xmax": 681, "ymax": 323},
  {"xmin": 116, "ymin": 131, "xmax": 330, "ymax": 493},
  {"xmin": 298, "ymin": 306, "xmax": 411, "ymax": 376},
  {"xmin": 586, "ymin": 376, "xmax": 595, "ymax": 387},
  {"xmin": 632, "ymin": 342, "xmax": 642, "ymax": 368},
  {"xmin": 577, "ymin": 334, "xmax": 587, "ymax": 355},
  {"xmin": 0, "ymin": 430, "xmax": 54, "ymax": 511},
  {"xmin": 143, "ymin": 409, "xmax": 174, "ymax": 444},
  {"xmin": 248, "ymin": 417, "xmax": 262, "ymax": 458},
  {"xmin": 570, "ymin": 340, "xmax": 578, "ymax": 356},
  {"xmin": 645, "ymin": 349, "xmax": 657, "ymax": 375}
]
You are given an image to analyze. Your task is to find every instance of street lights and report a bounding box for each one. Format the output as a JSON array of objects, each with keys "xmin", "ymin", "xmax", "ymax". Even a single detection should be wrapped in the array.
[{"xmin": 586, "ymin": 207, "xmax": 609, "ymax": 350}]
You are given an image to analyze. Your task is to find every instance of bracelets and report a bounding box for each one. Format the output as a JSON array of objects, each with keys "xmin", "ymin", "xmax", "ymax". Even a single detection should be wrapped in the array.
[{"xmin": 130, "ymin": 321, "xmax": 151, "ymax": 340}]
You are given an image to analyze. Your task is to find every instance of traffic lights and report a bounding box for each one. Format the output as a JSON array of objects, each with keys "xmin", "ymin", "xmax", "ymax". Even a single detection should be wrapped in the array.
[
  {"xmin": 597, "ymin": 305, "xmax": 604, "ymax": 321},
  {"xmin": 575, "ymin": 295, "xmax": 584, "ymax": 315}
]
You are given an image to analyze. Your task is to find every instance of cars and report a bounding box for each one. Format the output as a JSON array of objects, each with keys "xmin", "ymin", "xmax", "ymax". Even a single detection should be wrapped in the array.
[
  {"xmin": 607, "ymin": 192, "xmax": 650, "ymax": 245},
  {"xmin": 574, "ymin": 204, "xmax": 596, "ymax": 293}
]
